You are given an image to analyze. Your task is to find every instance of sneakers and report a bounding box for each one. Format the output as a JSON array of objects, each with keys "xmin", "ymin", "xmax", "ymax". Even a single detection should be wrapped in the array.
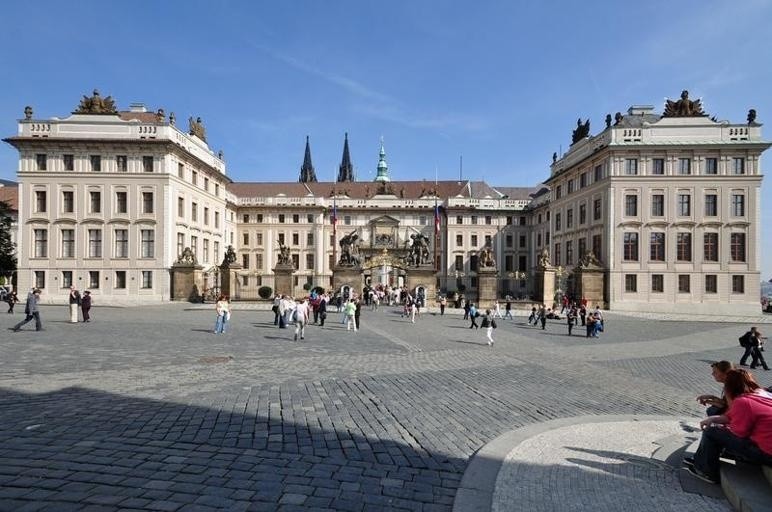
[
  {"xmin": 683, "ymin": 457, "xmax": 694, "ymax": 465},
  {"xmin": 688, "ymin": 465, "xmax": 717, "ymax": 484}
]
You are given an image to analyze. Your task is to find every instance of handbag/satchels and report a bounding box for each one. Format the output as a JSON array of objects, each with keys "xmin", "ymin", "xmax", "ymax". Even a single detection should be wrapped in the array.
[
  {"xmin": 475, "ymin": 311, "xmax": 479, "ymax": 316},
  {"xmin": 293, "ymin": 311, "xmax": 297, "ymax": 321},
  {"xmin": 492, "ymin": 319, "xmax": 498, "ymax": 328}
]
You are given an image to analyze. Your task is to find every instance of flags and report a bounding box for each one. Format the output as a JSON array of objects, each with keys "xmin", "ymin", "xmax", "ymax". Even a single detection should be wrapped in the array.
[
  {"xmin": 434, "ymin": 199, "xmax": 440, "ymax": 236},
  {"xmin": 333, "ymin": 198, "xmax": 338, "ymax": 227}
]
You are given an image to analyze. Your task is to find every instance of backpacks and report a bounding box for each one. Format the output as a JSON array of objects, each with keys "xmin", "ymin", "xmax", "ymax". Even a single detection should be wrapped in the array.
[{"xmin": 739, "ymin": 331, "xmax": 750, "ymax": 347}]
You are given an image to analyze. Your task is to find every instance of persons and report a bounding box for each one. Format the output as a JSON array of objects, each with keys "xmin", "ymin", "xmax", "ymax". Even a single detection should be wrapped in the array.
[
  {"xmin": 272, "ymin": 290, "xmax": 361, "ymax": 341},
  {"xmin": 88, "ymin": 89, "xmax": 104, "ymax": 112},
  {"xmin": 364, "ymin": 284, "xmax": 422, "ymax": 323},
  {"xmin": 438, "ymin": 288, "xmax": 478, "ymax": 329},
  {"xmin": 484, "ymin": 309, "xmax": 495, "ymax": 346},
  {"xmin": 528, "ymin": 304, "xmax": 560, "ymax": 329},
  {"xmin": 211, "ymin": 292, "xmax": 231, "ymax": 335},
  {"xmin": 679, "ymin": 324, "xmax": 771, "ymax": 488},
  {"xmin": 493, "ymin": 299, "xmax": 513, "ymax": 320},
  {"xmin": 6, "ymin": 286, "xmax": 92, "ymax": 331},
  {"xmin": 561, "ymin": 296, "xmax": 603, "ymax": 338},
  {"xmin": 676, "ymin": 90, "xmax": 692, "ymax": 116}
]
